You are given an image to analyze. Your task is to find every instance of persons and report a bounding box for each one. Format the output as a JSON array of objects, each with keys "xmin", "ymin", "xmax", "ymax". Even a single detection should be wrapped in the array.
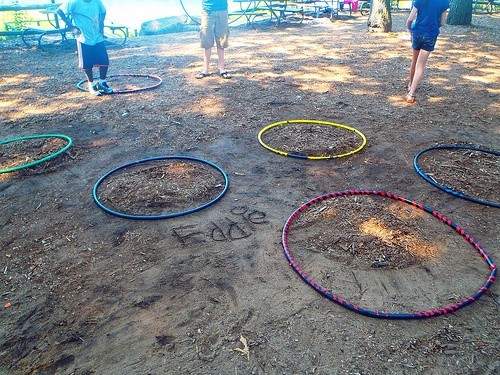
[
  {"xmin": 66, "ymin": 0, "xmax": 114, "ymax": 96},
  {"xmin": 196, "ymin": 0, "xmax": 232, "ymax": 78},
  {"xmin": 406, "ymin": 0, "xmax": 451, "ymax": 103}
]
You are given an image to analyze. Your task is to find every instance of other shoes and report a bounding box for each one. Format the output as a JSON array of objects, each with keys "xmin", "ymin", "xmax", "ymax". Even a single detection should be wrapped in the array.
[
  {"xmin": 406, "ymin": 94, "xmax": 415, "ymax": 103},
  {"xmin": 408, "ymin": 83, "xmax": 411, "ymax": 92}
]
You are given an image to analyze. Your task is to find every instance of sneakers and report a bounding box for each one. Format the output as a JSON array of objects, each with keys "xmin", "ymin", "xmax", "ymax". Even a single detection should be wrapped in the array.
[
  {"xmin": 89, "ymin": 85, "xmax": 101, "ymax": 96},
  {"xmin": 97, "ymin": 82, "xmax": 113, "ymax": 94}
]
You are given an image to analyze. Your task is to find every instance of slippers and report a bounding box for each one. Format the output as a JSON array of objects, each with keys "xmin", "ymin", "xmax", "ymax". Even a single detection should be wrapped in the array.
[
  {"xmin": 195, "ymin": 74, "xmax": 210, "ymax": 78},
  {"xmin": 220, "ymin": 72, "xmax": 232, "ymax": 79}
]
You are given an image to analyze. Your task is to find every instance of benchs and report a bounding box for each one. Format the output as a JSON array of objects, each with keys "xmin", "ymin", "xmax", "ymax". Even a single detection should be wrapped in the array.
[
  {"xmin": 0, "ymin": 24, "xmax": 130, "ymax": 52},
  {"xmin": 227, "ymin": 4, "xmax": 329, "ymax": 30}
]
